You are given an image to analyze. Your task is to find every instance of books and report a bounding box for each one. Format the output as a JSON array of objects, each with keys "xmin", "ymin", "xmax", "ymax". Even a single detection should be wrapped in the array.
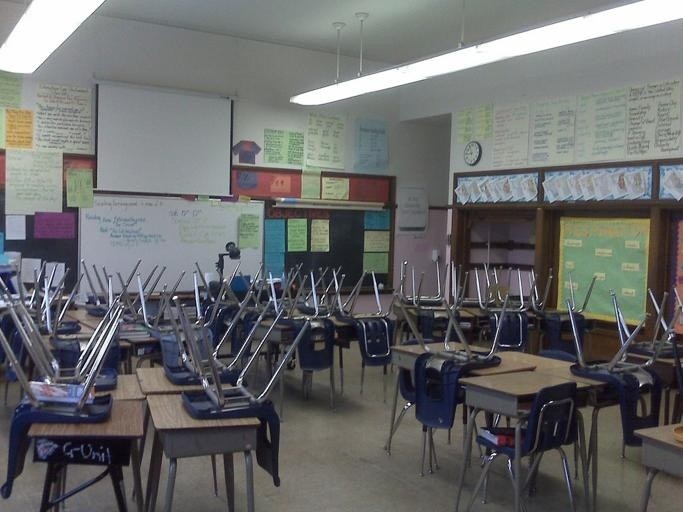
[
  {"xmin": 119, "ymin": 323, "xmax": 183, "ymax": 340},
  {"xmin": 266, "ymin": 278, "xmax": 282, "ymax": 285},
  {"xmin": 21, "ymin": 381, "xmax": 93, "ymax": 418},
  {"xmin": 479, "ymin": 426, "xmax": 525, "ymax": 447}
]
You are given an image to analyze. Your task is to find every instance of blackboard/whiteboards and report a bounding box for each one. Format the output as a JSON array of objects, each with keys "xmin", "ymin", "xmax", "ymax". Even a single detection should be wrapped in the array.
[
  {"xmin": 81, "ymin": 193, "xmax": 264, "ymax": 296},
  {"xmin": 264, "ymin": 197, "xmax": 391, "ymax": 292}
]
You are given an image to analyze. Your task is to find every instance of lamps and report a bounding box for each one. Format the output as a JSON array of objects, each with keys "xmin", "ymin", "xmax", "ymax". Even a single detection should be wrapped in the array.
[
  {"xmin": 288, "ymin": 0, "xmax": 683, "ymax": 109},
  {"xmin": 1, "ymin": 0, "xmax": 109, "ymax": 76}
]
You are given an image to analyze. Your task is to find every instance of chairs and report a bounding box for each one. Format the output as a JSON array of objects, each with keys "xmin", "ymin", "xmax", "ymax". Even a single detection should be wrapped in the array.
[
  {"xmin": 2, "ymin": 256, "xmax": 310, "ymax": 511},
  {"xmin": 385, "ymin": 256, "xmax": 683, "ymax": 511},
  {"xmin": 251, "ymin": 263, "xmax": 405, "ymax": 422}
]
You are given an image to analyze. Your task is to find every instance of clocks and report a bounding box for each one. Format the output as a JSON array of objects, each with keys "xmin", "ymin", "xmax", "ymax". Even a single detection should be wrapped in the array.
[{"xmin": 463, "ymin": 142, "xmax": 482, "ymax": 166}]
